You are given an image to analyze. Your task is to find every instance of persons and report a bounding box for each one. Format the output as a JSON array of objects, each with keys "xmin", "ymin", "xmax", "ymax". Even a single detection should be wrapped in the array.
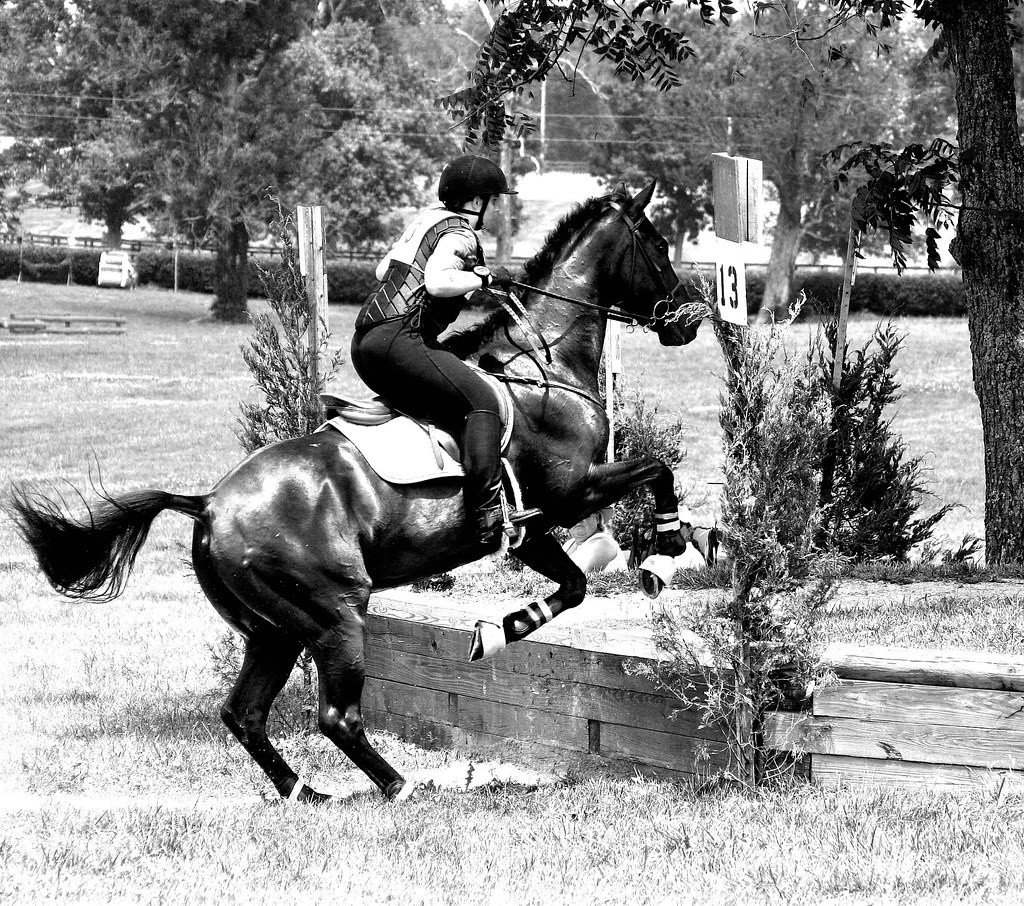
[{"xmin": 350, "ymin": 156, "xmax": 544, "ymax": 542}]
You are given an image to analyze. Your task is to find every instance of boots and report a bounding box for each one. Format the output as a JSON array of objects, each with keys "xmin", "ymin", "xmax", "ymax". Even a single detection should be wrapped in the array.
[{"xmin": 464, "ymin": 408, "xmax": 544, "ymax": 543}]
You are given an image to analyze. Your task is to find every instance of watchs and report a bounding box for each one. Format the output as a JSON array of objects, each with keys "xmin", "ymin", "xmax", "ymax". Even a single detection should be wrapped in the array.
[{"xmin": 471, "ymin": 265, "xmax": 491, "ymax": 288}]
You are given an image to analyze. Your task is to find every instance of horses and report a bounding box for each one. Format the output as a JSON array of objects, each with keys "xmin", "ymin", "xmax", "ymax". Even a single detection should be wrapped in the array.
[{"xmin": 2, "ymin": 178, "xmax": 708, "ymax": 804}]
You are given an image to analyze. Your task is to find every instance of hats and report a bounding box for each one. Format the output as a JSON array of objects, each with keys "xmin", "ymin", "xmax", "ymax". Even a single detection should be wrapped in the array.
[{"xmin": 437, "ymin": 154, "xmax": 519, "ymax": 200}]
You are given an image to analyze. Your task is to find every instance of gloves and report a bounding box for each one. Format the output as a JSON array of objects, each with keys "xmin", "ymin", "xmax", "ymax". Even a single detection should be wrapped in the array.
[
  {"xmin": 469, "ymin": 290, "xmax": 501, "ymax": 309},
  {"xmin": 490, "ymin": 266, "xmax": 512, "ymax": 294}
]
